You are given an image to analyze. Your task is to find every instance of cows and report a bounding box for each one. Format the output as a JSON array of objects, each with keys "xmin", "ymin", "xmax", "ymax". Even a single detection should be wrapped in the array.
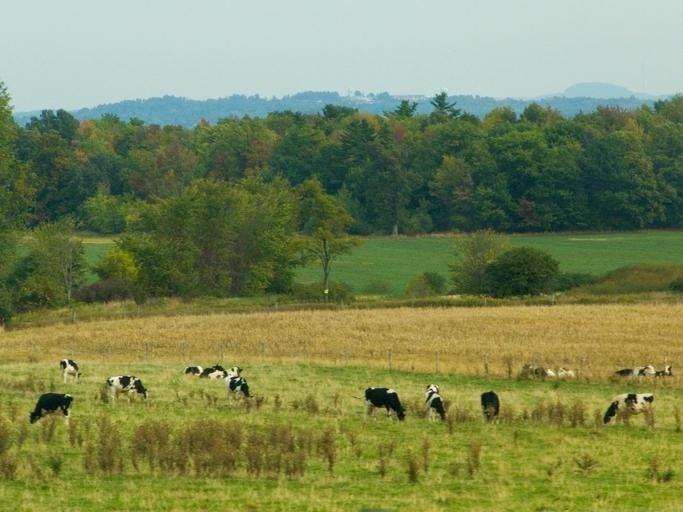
[
  {"xmin": 480, "ymin": 390, "xmax": 499, "ymax": 420},
  {"xmin": 616, "ymin": 364, "xmax": 672, "ymax": 379},
  {"xmin": 181, "ymin": 364, "xmax": 252, "ymax": 402},
  {"xmin": 104, "ymin": 373, "xmax": 149, "ymax": 402},
  {"xmin": 57, "ymin": 357, "xmax": 80, "ymax": 384},
  {"xmin": 364, "ymin": 387, "xmax": 404, "ymax": 422},
  {"xmin": 603, "ymin": 391, "xmax": 653, "ymax": 427},
  {"xmin": 421, "ymin": 382, "xmax": 446, "ymax": 423},
  {"xmin": 28, "ymin": 390, "xmax": 72, "ymax": 425}
]
